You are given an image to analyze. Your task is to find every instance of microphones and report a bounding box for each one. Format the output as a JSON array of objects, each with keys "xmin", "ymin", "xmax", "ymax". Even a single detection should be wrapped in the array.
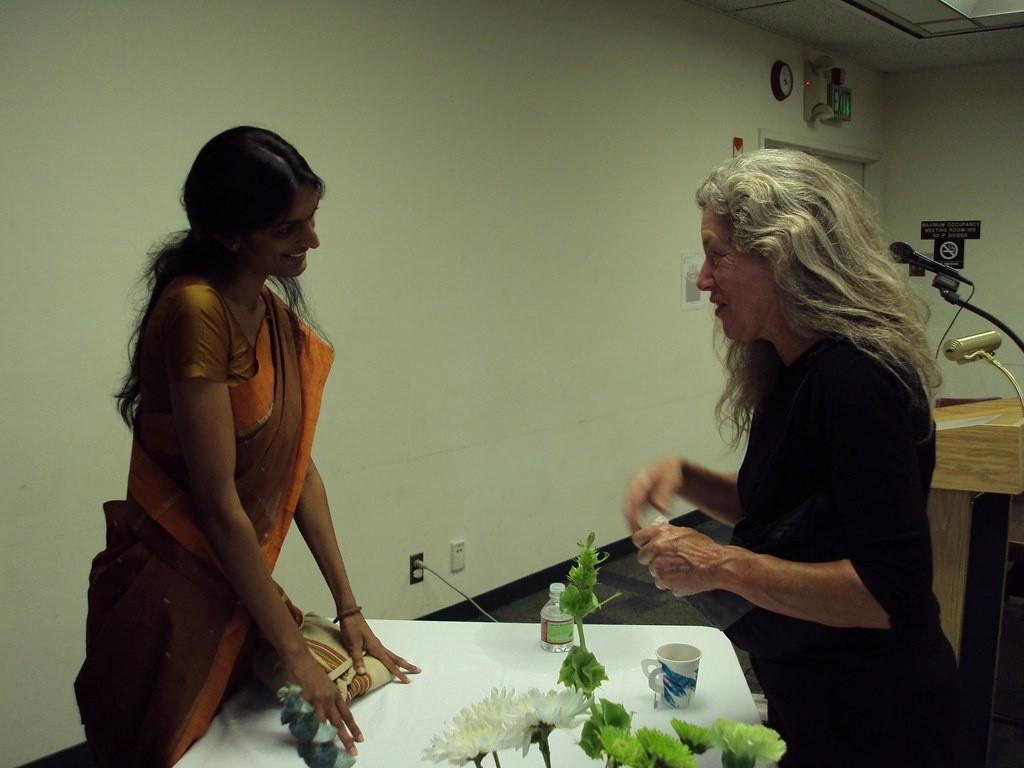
[{"xmin": 889, "ymin": 241, "xmax": 973, "ymax": 286}]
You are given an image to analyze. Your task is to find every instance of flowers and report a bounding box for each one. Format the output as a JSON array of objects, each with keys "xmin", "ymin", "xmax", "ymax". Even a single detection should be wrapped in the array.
[{"xmin": 276, "ymin": 533, "xmax": 787, "ymax": 767}]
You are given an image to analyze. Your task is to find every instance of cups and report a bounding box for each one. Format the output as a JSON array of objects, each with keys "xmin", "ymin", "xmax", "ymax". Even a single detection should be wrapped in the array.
[{"xmin": 640, "ymin": 642, "xmax": 703, "ymax": 710}]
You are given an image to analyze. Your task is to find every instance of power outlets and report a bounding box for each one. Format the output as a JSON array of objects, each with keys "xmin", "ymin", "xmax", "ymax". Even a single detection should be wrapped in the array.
[
  {"xmin": 409, "ymin": 552, "xmax": 424, "ymax": 584},
  {"xmin": 450, "ymin": 541, "xmax": 466, "ymax": 571}
]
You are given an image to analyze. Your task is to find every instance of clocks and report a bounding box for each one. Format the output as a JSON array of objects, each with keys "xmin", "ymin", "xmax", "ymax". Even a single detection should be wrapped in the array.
[{"xmin": 770, "ymin": 60, "xmax": 793, "ymax": 101}]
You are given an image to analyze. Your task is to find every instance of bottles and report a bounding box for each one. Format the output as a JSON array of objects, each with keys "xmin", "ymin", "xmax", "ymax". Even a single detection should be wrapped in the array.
[{"xmin": 540, "ymin": 583, "xmax": 576, "ymax": 653}]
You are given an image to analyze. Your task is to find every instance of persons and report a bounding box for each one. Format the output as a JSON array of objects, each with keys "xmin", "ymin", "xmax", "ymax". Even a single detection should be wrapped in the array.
[
  {"xmin": 73, "ymin": 125, "xmax": 419, "ymax": 767},
  {"xmin": 621, "ymin": 149, "xmax": 963, "ymax": 768}
]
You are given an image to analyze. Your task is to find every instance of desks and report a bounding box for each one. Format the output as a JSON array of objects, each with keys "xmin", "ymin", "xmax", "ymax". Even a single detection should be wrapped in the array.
[
  {"xmin": 172, "ymin": 614, "xmax": 778, "ymax": 768},
  {"xmin": 922, "ymin": 396, "xmax": 1024, "ymax": 768}
]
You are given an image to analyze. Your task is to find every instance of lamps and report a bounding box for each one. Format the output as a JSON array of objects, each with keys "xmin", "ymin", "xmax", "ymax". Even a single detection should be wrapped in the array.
[
  {"xmin": 803, "ymin": 57, "xmax": 835, "ymax": 123},
  {"xmin": 944, "ymin": 331, "xmax": 1024, "ymax": 416}
]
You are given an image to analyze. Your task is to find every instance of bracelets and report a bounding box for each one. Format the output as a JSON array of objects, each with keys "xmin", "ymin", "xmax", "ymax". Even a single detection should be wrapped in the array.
[{"xmin": 335, "ymin": 607, "xmax": 360, "ymax": 623}]
